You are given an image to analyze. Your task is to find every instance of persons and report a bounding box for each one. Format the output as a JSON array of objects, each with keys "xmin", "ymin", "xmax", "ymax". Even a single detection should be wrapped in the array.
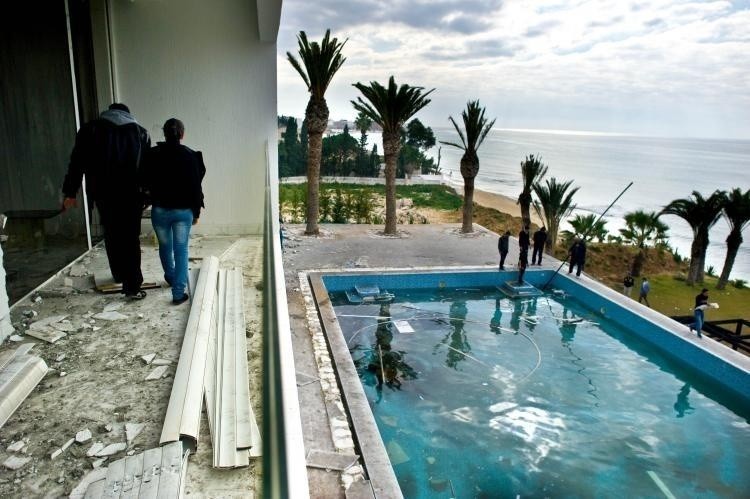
[
  {"xmin": 518, "ymin": 226, "xmax": 547, "ymax": 283},
  {"xmin": 688, "ymin": 288, "xmax": 710, "ymax": 338},
  {"xmin": 624, "ymin": 272, "xmax": 634, "ymax": 297},
  {"xmin": 568, "ymin": 236, "xmax": 586, "ymax": 276},
  {"xmin": 498, "ymin": 231, "xmax": 511, "ymax": 270},
  {"xmin": 62, "ymin": 103, "xmax": 206, "ymax": 305},
  {"xmin": 639, "ymin": 277, "xmax": 650, "ymax": 307}
]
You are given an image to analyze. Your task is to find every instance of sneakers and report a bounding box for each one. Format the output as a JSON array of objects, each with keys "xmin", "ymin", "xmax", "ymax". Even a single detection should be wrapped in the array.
[
  {"xmin": 164, "ymin": 273, "xmax": 189, "ymax": 306},
  {"xmin": 530, "ymin": 262, "xmax": 542, "ymax": 267},
  {"xmin": 688, "ymin": 323, "xmax": 703, "ymax": 339},
  {"xmin": 566, "ymin": 271, "xmax": 581, "ymax": 279},
  {"xmin": 114, "ymin": 276, "xmax": 147, "ymax": 300},
  {"xmin": 499, "ymin": 265, "xmax": 505, "ymax": 270}
]
